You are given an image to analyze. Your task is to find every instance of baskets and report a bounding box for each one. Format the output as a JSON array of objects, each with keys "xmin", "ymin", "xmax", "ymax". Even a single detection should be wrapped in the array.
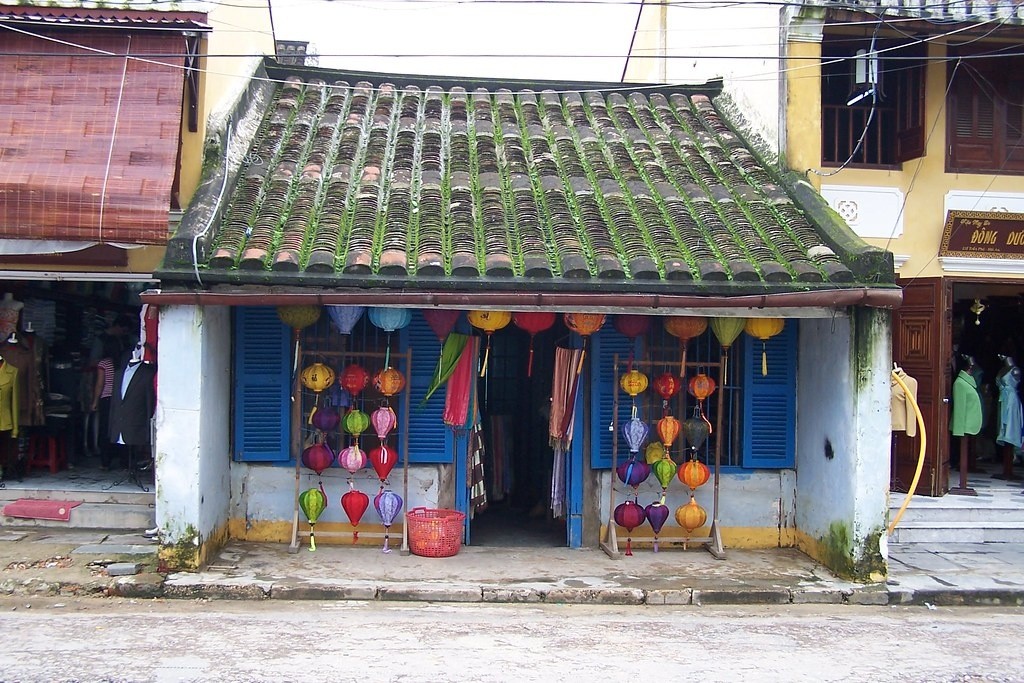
[{"xmin": 406, "ymin": 506, "xmax": 466, "ymax": 557}]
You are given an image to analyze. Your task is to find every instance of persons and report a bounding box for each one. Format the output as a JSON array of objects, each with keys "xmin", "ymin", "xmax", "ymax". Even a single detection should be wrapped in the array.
[
  {"xmin": 891, "ymin": 367, "xmax": 918, "ymax": 438},
  {"xmin": 72, "ymin": 335, "xmax": 103, "ymax": 457},
  {"xmin": 0, "ymin": 292, "xmax": 46, "ymax": 437},
  {"xmin": 996, "ymin": 345, "xmax": 1024, "ymax": 455},
  {"xmin": 949, "ymin": 354, "xmax": 983, "ymax": 436},
  {"xmin": 90, "ymin": 336, "xmax": 121, "ymax": 470},
  {"xmin": 107, "ymin": 303, "xmax": 159, "ymax": 471}
]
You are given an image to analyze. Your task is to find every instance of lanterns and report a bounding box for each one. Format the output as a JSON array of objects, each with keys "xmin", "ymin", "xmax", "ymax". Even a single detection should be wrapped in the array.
[
  {"xmin": 298, "ymin": 363, "xmax": 405, "ymax": 552},
  {"xmin": 279, "ymin": 306, "xmax": 784, "ymax": 385},
  {"xmin": 614, "ymin": 368, "xmax": 716, "ymax": 556}
]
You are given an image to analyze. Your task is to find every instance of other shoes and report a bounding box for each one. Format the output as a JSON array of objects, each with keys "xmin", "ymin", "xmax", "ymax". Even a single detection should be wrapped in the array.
[{"xmin": 99, "ymin": 465, "xmax": 109, "ymax": 470}]
